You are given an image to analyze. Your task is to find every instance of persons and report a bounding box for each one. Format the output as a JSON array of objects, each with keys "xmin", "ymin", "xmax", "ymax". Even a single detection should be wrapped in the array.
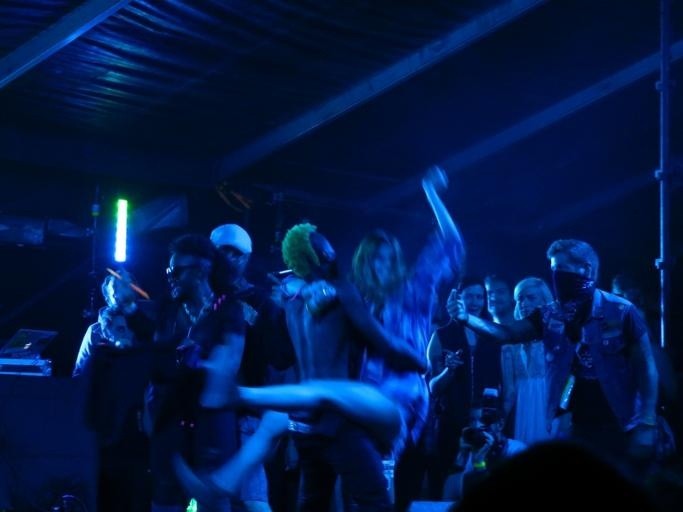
[
  {"xmin": 211, "ymin": 224, "xmax": 285, "ymax": 511},
  {"xmin": 501, "ymin": 276, "xmax": 571, "ymax": 446},
  {"xmin": 427, "ymin": 278, "xmax": 500, "ymax": 442},
  {"xmin": 444, "ymin": 240, "xmax": 657, "ymax": 433},
  {"xmin": 267, "ymin": 224, "xmax": 432, "ymax": 512},
  {"xmin": 175, "ymin": 165, "xmax": 462, "ymax": 504},
  {"xmin": 479, "ymin": 272, "xmax": 515, "ymax": 385},
  {"xmin": 74, "ymin": 270, "xmax": 157, "ymax": 438},
  {"xmin": 163, "ymin": 236, "xmax": 246, "ymax": 511}
]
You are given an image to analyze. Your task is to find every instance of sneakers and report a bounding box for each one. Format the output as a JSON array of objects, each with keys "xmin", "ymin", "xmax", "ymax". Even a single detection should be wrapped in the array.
[
  {"xmin": 199, "ymin": 334, "xmax": 246, "ymax": 409},
  {"xmin": 169, "ymin": 452, "xmax": 233, "ymax": 511}
]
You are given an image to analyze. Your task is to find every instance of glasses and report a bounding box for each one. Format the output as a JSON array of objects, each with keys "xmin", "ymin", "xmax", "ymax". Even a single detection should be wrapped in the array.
[{"xmin": 165, "ymin": 264, "xmax": 202, "ymax": 277}]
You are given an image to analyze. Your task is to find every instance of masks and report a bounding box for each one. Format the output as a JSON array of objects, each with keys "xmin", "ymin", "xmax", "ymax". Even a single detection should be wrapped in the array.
[{"xmin": 551, "ymin": 271, "xmax": 595, "ymax": 304}]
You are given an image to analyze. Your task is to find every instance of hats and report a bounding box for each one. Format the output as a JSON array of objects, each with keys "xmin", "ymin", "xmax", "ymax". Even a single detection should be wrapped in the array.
[{"xmin": 211, "ymin": 224, "xmax": 253, "ymax": 255}]
